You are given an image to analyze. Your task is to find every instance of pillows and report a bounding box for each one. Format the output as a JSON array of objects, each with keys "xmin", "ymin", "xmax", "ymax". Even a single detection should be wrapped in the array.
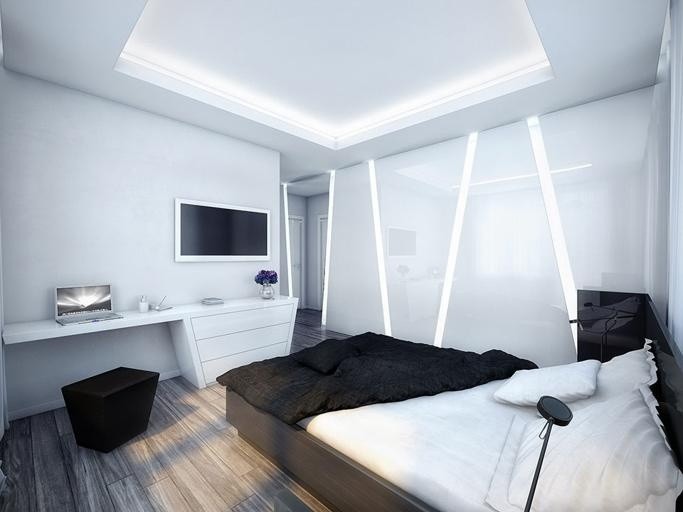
[{"xmin": 489, "ymin": 337, "xmax": 683, "ymax": 512}]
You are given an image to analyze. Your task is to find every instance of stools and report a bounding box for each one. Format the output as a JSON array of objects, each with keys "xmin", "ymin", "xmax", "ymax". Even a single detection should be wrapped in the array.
[{"xmin": 61, "ymin": 367, "xmax": 160, "ymax": 453}]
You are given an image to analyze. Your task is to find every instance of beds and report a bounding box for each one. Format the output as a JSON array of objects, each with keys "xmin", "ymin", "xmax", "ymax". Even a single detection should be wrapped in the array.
[{"xmin": 215, "ymin": 289, "xmax": 683, "ymax": 512}]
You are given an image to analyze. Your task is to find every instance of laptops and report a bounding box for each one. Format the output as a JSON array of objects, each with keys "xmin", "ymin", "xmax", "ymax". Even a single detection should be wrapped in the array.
[{"xmin": 55, "ymin": 284, "xmax": 123, "ymax": 326}]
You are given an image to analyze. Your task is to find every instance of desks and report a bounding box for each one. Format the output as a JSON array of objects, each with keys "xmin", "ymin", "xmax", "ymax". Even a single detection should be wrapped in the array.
[{"xmin": 2, "ymin": 293, "xmax": 300, "ymax": 389}]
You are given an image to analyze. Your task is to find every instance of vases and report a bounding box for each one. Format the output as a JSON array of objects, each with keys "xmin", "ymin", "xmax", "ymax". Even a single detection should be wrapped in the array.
[{"xmin": 258, "ymin": 284, "xmax": 275, "ymax": 300}]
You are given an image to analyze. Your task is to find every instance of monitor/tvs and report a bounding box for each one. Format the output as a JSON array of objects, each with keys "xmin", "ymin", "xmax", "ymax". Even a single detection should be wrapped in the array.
[{"xmin": 175, "ymin": 197, "xmax": 270, "ymax": 263}]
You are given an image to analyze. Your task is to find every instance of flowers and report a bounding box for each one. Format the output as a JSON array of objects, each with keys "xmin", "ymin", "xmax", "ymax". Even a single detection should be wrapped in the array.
[{"xmin": 254, "ymin": 269, "xmax": 279, "ymax": 284}]
